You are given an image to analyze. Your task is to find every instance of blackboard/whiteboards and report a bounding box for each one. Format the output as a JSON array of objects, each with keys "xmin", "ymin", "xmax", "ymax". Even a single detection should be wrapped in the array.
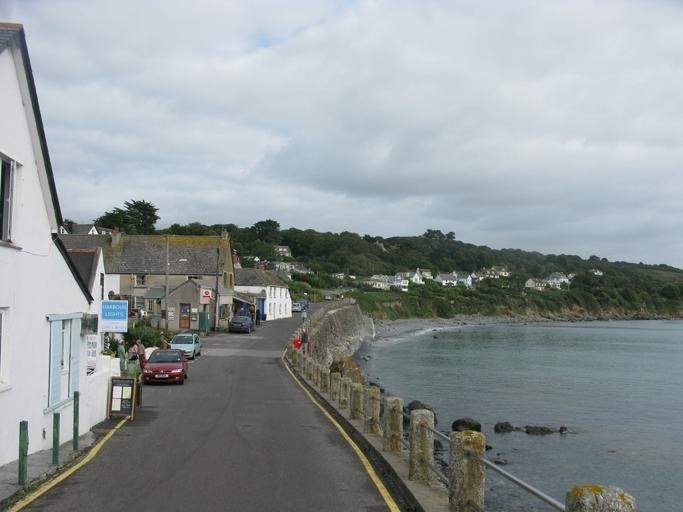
[{"xmin": 107, "ymin": 376, "xmax": 136, "ymax": 416}]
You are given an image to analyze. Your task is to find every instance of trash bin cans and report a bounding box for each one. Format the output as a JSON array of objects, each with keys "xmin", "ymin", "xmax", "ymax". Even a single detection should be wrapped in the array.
[{"xmin": 136, "ymin": 381, "xmax": 143, "ymax": 408}]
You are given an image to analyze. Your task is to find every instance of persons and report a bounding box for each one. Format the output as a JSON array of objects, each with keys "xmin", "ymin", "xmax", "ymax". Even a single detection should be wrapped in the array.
[
  {"xmin": 240, "ymin": 305, "xmax": 261, "ymax": 327},
  {"xmin": 301, "ymin": 309, "xmax": 307, "ymax": 324},
  {"xmin": 104, "ymin": 331, "xmax": 171, "ymax": 372}
]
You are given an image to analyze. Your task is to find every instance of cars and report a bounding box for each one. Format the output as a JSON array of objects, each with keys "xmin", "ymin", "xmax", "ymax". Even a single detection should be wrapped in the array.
[
  {"xmin": 229, "ymin": 316, "xmax": 256, "ymax": 334},
  {"xmin": 167, "ymin": 333, "xmax": 201, "ymax": 360},
  {"xmin": 142, "ymin": 349, "xmax": 188, "ymax": 386},
  {"xmin": 292, "ymin": 301, "xmax": 307, "ymax": 312}
]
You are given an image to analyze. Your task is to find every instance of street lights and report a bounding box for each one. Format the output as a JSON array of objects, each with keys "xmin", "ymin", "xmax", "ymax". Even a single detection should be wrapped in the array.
[{"xmin": 165, "ymin": 236, "xmax": 188, "ymax": 340}]
